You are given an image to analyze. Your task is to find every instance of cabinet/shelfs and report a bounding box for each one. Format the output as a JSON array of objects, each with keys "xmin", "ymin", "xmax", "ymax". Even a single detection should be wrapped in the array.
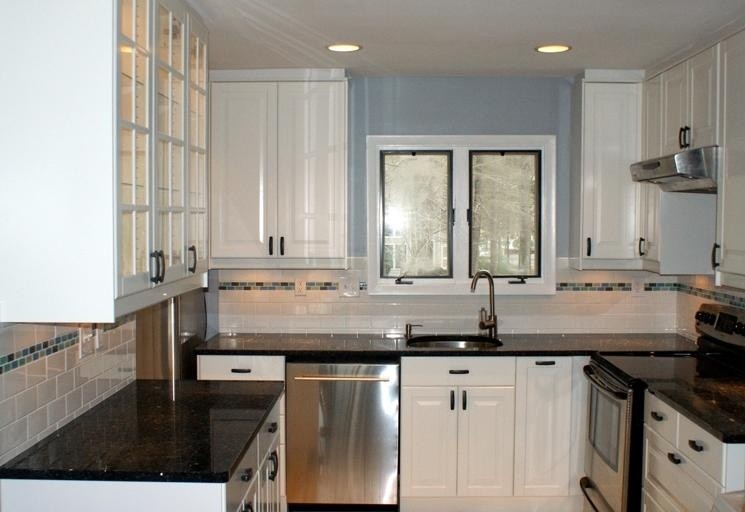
[
  {"xmin": 41, "ymin": 416, "xmax": 259, "ymax": 511},
  {"xmin": 517, "ymin": 357, "xmax": 590, "ymax": 495},
  {"xmin": 708, "ymin": 30, "xmax": 745, "ymax": 288},
  {"xmin": 0, "ymin": 2, "xmax": 188, "ymax": 326},
  {"xmin": 661, "ymin": 41, "xmax": 724, "ymax": 164},
  {"xmin": 638, "ymin": 73, "xmax": 717, "ymax": 273},
  {"xmin": 569, "ymin": 82, "xmax": 644, "ymax": 268},
  {"xmin": 260, "ymin": 390, "xmax": 288, "ymax": 512},
  {"xmin": 187, "ymin": 32, "xmax": 214, "ymax": 284},
  {"xmin": 197, "ymin": 354, "xmax": 286, "ymax": 382},
  {"xmin": 398, "ymin": 357, "xmax": 514, "ymax": 498},
  {"xmin": 640, "ymin": 390, "xmax": 745, "ymax": 511},
  {"xmin": 210, "ymin": 77, "xmax": 349, "ymax": 261}
]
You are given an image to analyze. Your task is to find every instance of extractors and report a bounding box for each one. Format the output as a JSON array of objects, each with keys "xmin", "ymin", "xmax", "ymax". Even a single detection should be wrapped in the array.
[{"xmin": 628, "ymin": 146, "xmax": 717, "ymax": 198}]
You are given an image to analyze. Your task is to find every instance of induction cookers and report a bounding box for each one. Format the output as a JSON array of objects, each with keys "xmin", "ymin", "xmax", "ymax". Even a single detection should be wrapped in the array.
[{"xmin": 591, "ymin": 349, "xmax": 745, "ymax": 385}]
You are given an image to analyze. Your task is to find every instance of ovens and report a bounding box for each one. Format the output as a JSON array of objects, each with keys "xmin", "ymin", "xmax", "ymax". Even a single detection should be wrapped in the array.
[{"xmin": 577, "ymin": 361, "xmax": 644, "ymax": 511}]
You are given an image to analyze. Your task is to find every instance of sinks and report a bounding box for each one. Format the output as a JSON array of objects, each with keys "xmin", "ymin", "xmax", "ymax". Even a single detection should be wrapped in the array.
[{"xmin": 408, "ymin": 334, "xmax": 503, "ymax": 349}]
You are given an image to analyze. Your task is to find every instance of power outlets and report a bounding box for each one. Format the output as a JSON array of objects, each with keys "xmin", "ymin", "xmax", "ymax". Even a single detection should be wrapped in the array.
[{"xmin": 294, "ymin": 278, "xmax": 306, "ymax": 296}]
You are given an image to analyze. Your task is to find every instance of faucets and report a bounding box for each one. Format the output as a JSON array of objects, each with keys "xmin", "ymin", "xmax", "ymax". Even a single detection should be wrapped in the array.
[{"xmin": 472, "ymin": 270, "xmax": 497, "ymax": 338}]
